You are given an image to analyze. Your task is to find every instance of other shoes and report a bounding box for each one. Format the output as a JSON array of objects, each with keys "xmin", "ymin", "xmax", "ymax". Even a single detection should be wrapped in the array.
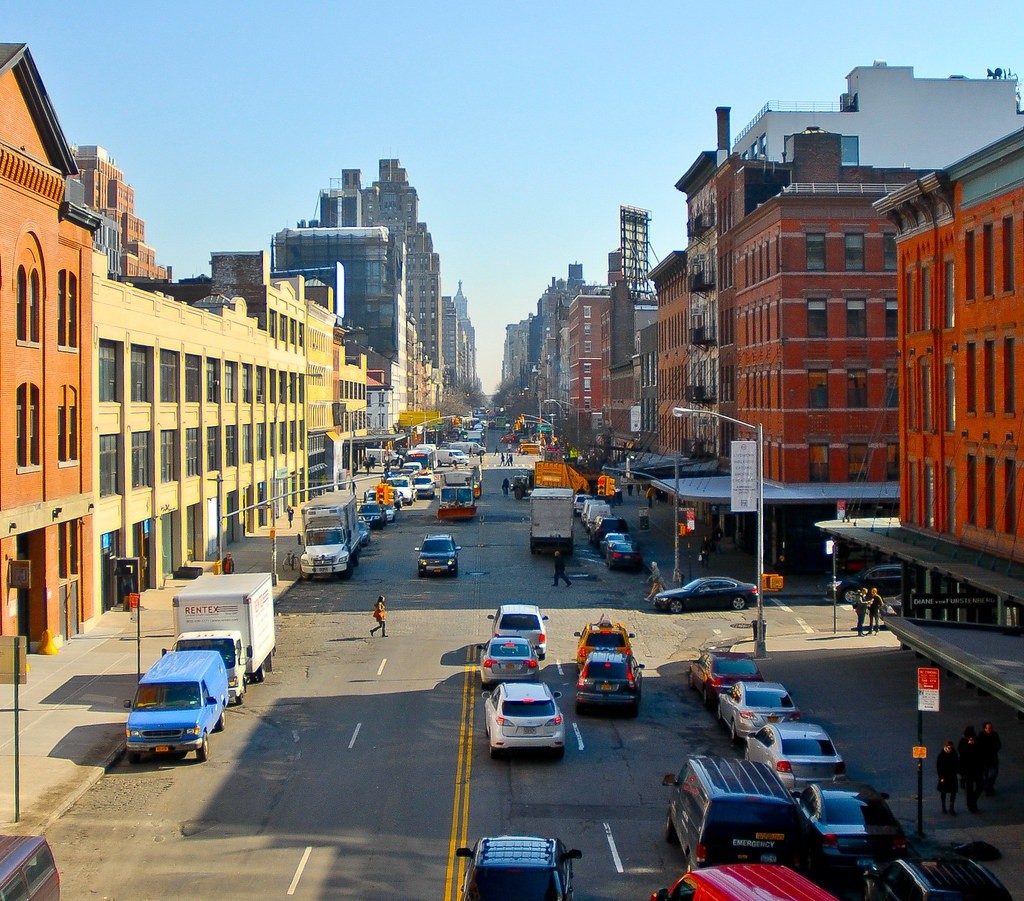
[
  {"xmin": 566, "ymin": 582, "xmax": 572, "ymax": 587},
  {"xmin": 644, "ymin": 598, "xmax": 651, "ymax": 602},
  {"xmin": 370, "ymin": 630, "xmax": 374, "ymax": 636},
  {"xmin": 858, "ymin": 633, "xmax": 867, "ymax": 637},
  {"xmin": 382, "ymin": 635, "xmax": 388, "ymax": 637},
  {"xmin": 960, "ymin": 779, "xmax": 966, "ymax": 789},
  {"xmin": 552, "ymin": 584, "xmax": 558, "ymax": 586}
]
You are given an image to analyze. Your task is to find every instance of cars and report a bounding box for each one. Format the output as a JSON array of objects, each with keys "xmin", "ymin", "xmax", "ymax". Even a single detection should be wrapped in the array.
[
  {"xmin": 454, "ymin": 601, "xmax": 1016, "ymax": 901},
  {"xmin": 827, "ymin": 564, "xmax": 916, "ymax": 603},
  {"xmin": 414, "ymin": 534, "xmax": 461, "ymax": 578},
  {"xmin": 367, "ymin": 460, "xmax": 435, "ymax": 522},
  {"xmin": 655, "ymin": 576, "xmax": 759, "ymax": 613},
  {"xmin": 573, "ymin": 493, "xmax": 642, "ymax": 570},
  {"xmin": 357, "ymin": 503, "xmax": 387, "ymax": 530},
  {"xmin": 358, "ymin": 521, "xmax": 371, "ymax": 546}
]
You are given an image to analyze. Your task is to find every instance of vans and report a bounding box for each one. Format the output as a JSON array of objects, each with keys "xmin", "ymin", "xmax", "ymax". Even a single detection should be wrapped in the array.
[
  {"xmin": 123, "ymin": 649, "xmax": 230, "ymax": 762},
  {"xmin": 367, "ymin": 450, "xmax": 399, "ymax": 467},
  {"xmin": 521, "ymin": 444, "xmax": 545, "ymax": 455},
  {"xmin": 437, "ymin": 450, "xmax": 469, "ymax": 467},
  {"xmin": 448, "ymin": 442, "xmax": 487, "ymax": 456}
]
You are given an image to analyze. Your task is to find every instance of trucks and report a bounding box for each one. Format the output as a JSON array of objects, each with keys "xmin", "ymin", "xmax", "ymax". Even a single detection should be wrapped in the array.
[
  {"xmin": 297, "ymin": 494, "xmax": 361, "ymax": 580},
  {"xmin": 510, "ymin": 461, "xmax": 600, "ymax": 500},
  {"xmin": 171, "ymin": 572, "xmax": 277, "ymax": 706},
  {"xmin": 530, "ymin": 487, "xmax": 576, "ymax": 554}
]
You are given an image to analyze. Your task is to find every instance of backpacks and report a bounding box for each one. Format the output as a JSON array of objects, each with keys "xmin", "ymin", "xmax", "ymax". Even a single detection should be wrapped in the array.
[{"xmin": 852, "ymin": 595, "xmax": 864, "ymax": 609}]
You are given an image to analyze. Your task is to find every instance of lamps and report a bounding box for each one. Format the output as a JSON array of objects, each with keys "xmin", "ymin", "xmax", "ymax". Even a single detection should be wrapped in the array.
[
  {"xmin": 88, "ymin": 504, "xmax": 94, "ymax": 512},
  {"xmin": 9, "ymin": 523, "xmax": 17, "ymax": 534},
  {"xmin": 52, "ymin": 508, "xmax": 62, "ymax": 521}
]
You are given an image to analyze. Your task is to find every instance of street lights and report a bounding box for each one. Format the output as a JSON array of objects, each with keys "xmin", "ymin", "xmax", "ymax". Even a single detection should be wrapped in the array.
[
  {"xmin": 673, "ymin": 406, "xmax": 766, "ymax": 659},
  {"xmin": 270, "ymin": 374, "xmax": 323, "ymax": 587},
  {"xmin": 350, "ymin": 402, "xmax": 390, "ymax": 495}
]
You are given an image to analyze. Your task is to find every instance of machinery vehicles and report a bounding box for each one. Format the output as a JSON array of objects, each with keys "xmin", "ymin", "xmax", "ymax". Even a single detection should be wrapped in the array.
[{"xmin": 437, "ymin": 471, "xmax": 477, "ymax": 519}]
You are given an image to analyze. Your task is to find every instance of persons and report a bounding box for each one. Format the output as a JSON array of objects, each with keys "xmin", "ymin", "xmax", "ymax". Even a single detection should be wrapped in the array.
[
  {"xmin": 469, "ymin": 446, "xmax": 513, "ymax": 466},
  {"xmin": 352, "ymin": 454, "xmax": 376, "ymax": 476},
  {"xmin": 222, "ymin": 552, "xmax": 235, "ymax": 575},
  {"xmin": 699, "ymin": 523, "xmax": 723, "ymax": 567},
  {"xmin": 936, "ymin": 722, "xmax": 1002, "ymax": 817},
  {"xmin": 287, "ymin": 505, "xmax": 294, "ymax": 528},
  {"xmin": 551, "ymin": 551, "xmax": 572, "ymax": 587},
  {"xmin": 501, "ymin": 477, "xmax": 509, "ymax": 498},
  {"xmin": 856, "ymin": 588, "xmax": 884, "ymax": 637},
  {"xmin": 370, "ymin": 595, "xmax": 388, "ymax": 637},
  {"xmin": 627, "ymin": 484, "xmax": 656, "ymax": 509},
  {"xmin": 643, "ymin": 562, "xmax": 665, "ymax": 603},
  {"xmin": 773, "ymin": 556, "xmax": 784, "ymax": 576},
  {"xmin": 348, "ymin": 480, "xmax": 356, "ymax": 495},
  {"xmin": 532, "ymin": 432, "xmax": 559, "ymax": 452}
]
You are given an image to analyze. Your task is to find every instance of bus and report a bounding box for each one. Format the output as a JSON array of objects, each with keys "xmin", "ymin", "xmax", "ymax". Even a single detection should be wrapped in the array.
[{"xmin": 405, "ymin": 447, "xmax": 434, "ymax": 471}]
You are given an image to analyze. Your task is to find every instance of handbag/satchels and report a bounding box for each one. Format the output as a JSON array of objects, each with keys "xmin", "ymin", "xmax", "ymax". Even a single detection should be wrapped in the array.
[{"xmin": 373, "ymin": 608, "xmax": 385, "ymax": 621}]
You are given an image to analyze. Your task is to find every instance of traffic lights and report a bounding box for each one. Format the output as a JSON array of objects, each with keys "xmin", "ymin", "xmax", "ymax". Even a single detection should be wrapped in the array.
[
  {"xmin": 377, "ymin": 486, "xmax": 386, "ymax": 505},
  {"xmin": 385, "ymin": 487, "xmax": 395, "ymax": 505},
  {"xmin": 598, "ymin": 477, "xmax": 605, "ymax": 496},
  {"xmin": 606, "ymin": 478, "xmax": 616, "ymax": 496}
]
